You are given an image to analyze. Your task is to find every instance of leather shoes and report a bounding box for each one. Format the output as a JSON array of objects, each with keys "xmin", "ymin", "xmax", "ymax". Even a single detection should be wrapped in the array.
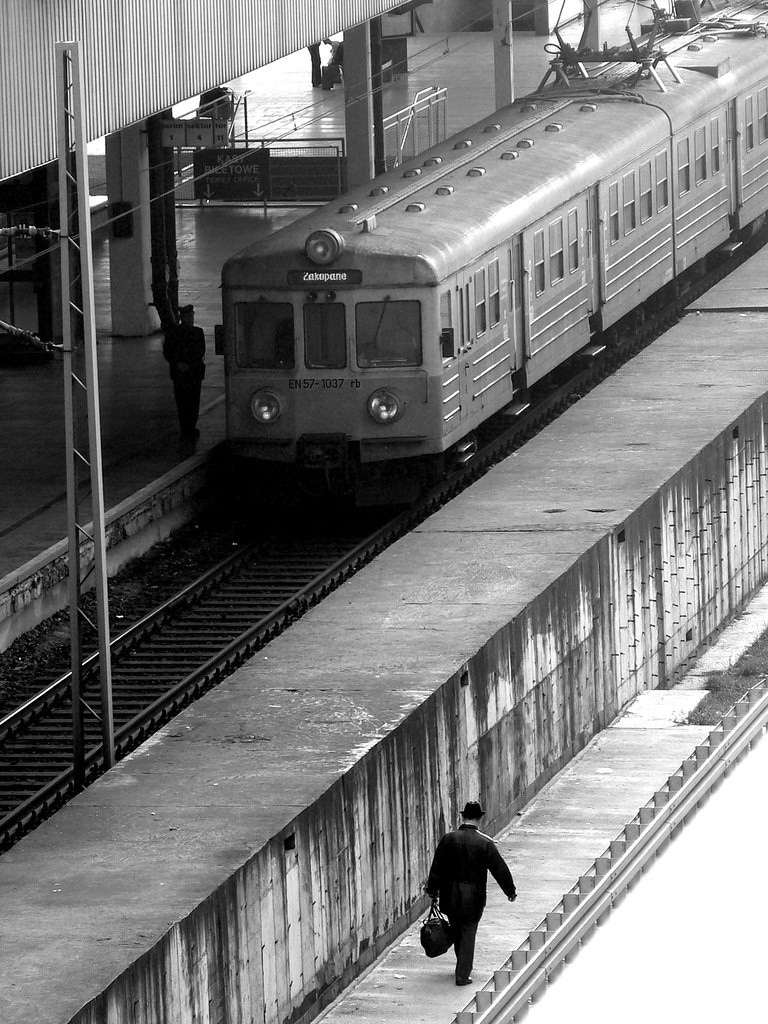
[{"xmin": 455, "ymin": 976, "xmax": 473, "ymax": 987}]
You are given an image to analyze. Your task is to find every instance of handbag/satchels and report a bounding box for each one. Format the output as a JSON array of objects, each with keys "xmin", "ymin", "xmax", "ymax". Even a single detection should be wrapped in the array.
[{"xmin": 421, "ymin": 902, "xmax": 454, "ymax": 958}]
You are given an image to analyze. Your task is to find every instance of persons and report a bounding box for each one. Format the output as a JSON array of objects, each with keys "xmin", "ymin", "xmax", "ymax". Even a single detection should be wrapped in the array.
[
  {"xmin": 424, "ymin": 801, "xmax": 517, "ymax": 986},
  {"xmin": 161, "ymin": 305, "xmax": 206, "ymax": 450},
  {"xmin": 307, "ymin": 38, "xmax": 334, "ymax": 90}
]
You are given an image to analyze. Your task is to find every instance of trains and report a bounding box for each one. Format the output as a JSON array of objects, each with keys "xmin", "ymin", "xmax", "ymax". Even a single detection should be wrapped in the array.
[{"xmin": 213, "ymin": 0, "xmax": 767, "ymax": 481}]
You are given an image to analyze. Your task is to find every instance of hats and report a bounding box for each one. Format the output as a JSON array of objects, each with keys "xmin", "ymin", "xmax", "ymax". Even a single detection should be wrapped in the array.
[
  {"xmin": 179, "ymin": 305, "xmax": 193, "ymax": 314},
  {"xmin": 459, "ymin": 801, "xmax": 486, "ymax": 815}
]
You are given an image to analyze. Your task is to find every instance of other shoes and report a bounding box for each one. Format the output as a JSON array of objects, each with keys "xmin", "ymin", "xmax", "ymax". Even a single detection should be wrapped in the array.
[{"xmin": 184, "ymin": 429, "xmax": 200, "ymax": 437}]
